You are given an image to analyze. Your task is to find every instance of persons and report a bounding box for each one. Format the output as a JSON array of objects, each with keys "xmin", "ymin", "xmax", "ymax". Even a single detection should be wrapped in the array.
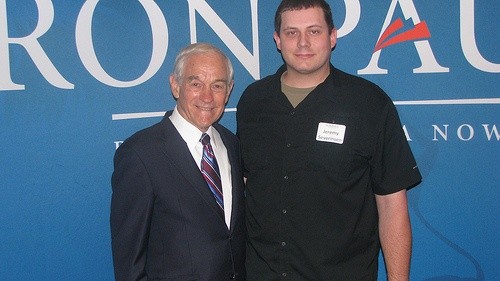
[
  {"xmin": 236, "ymin": 0, "xmax": 422, "ymax": 281},
  {"xmin": 110, "ymin": 42, "xmax": 246, "ymax": 281}
]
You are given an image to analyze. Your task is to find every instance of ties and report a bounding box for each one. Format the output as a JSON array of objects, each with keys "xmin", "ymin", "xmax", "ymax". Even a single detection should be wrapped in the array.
[{"xmin": 199, "ymin": 133, "xmax": 225, "ymax": 221}]
